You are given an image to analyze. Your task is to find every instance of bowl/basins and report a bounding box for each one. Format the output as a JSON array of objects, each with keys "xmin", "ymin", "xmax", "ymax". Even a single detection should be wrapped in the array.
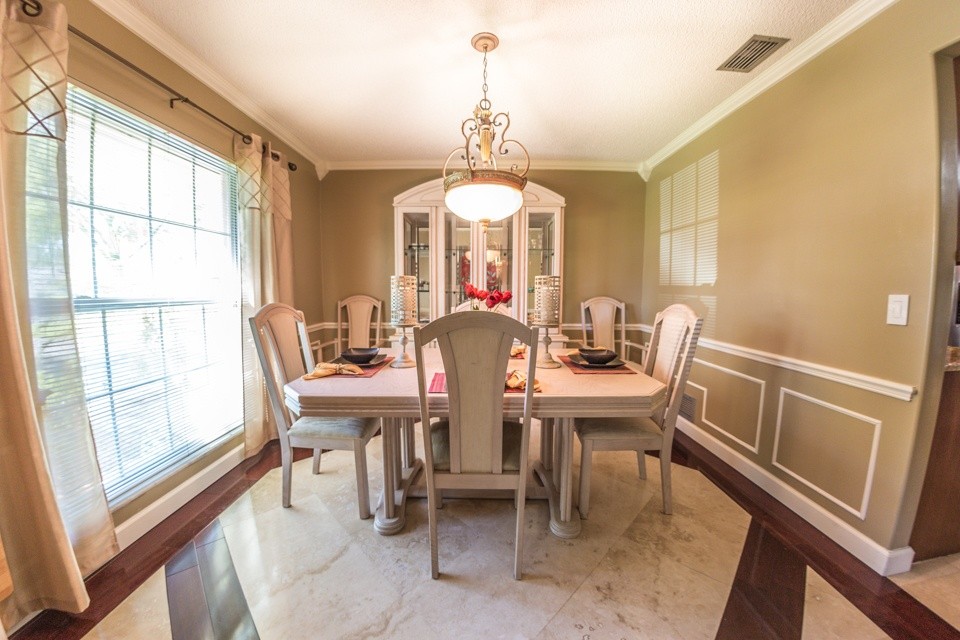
[
  {"xmin": 578, "ymin": 349, "xmax": 617, "ymax": 363},
  {"xmin": 341, "ymin": 348, "xmax": 379, "ymax": 362}
]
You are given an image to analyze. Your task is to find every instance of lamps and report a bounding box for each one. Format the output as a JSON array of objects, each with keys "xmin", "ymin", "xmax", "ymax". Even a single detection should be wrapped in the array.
[
  {"xmin": 465, "ymin": 238, "xmax": 500, "ymax": 263},
  {"xmin": 390, "ymin": 275, "xmax": 418, "ymax": 368},
  {"xmin": 443, "ymin": 33, "xmax": 531, "ymax": 233},
  {"xmin": 535, "ymin": 275, "xmax": 561, "ymax": 369}
]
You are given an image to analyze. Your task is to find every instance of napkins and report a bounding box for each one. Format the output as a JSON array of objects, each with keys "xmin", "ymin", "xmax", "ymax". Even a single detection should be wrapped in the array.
[
  {"xmin": 511, "ymin": 346, "xmax": 525, "ymax": 356},
  {"xmin": 507, "ymin": 370, "xmax": 540, "ymax": 390},
  {"xmin": 304, "ymin": 362, "xmax": 364, "ymax": 380}
]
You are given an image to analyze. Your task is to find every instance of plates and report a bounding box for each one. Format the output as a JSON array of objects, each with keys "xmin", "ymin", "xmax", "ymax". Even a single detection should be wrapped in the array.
[
  {"xmin": 331, "ymin": 355, "xmax": 387, "ymax": 368},
  {"xmin": 568, "ymin": 355, "xmax": 626, "ymax": 368}
]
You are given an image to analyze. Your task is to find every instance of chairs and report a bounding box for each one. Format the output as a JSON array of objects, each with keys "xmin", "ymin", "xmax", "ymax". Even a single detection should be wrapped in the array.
[{"xmin": 248, "ymin": 295, "xmax": 704, "ymax": 580}]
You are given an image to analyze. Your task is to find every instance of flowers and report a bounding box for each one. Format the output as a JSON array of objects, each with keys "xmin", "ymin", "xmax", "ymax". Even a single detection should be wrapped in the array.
[
  {"xmin": 463, "ymin": 282, "xmax": 513, "ymax": 310},
  {"xmin": 460, "ymin": 256, "xmax": 498, "ymax": 290}
]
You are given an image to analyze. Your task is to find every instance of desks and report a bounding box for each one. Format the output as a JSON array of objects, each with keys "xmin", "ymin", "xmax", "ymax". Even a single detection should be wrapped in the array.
[{"xmin": 283, "ymin": 349, "xmax": 668, "ymax": 539}]
